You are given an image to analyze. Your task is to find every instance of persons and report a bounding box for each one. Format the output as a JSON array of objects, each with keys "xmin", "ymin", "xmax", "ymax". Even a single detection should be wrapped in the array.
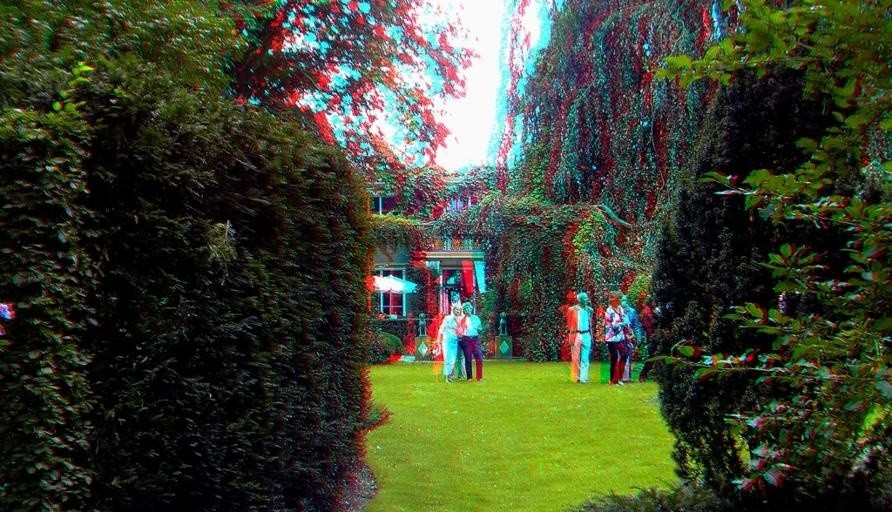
[
  {"xmin": 560, "ymin": 288, "xmax": 660, "ymax": 385},
  {"xmin": 436, "ymin": 301, "xmax": 486, "ymax": 383}
]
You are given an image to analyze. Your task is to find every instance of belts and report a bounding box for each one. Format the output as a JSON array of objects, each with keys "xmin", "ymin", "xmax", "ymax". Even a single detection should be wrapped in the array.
[{"xmin": 576, "ymin": 330, "xmax": 590, "ymax": 334}]
